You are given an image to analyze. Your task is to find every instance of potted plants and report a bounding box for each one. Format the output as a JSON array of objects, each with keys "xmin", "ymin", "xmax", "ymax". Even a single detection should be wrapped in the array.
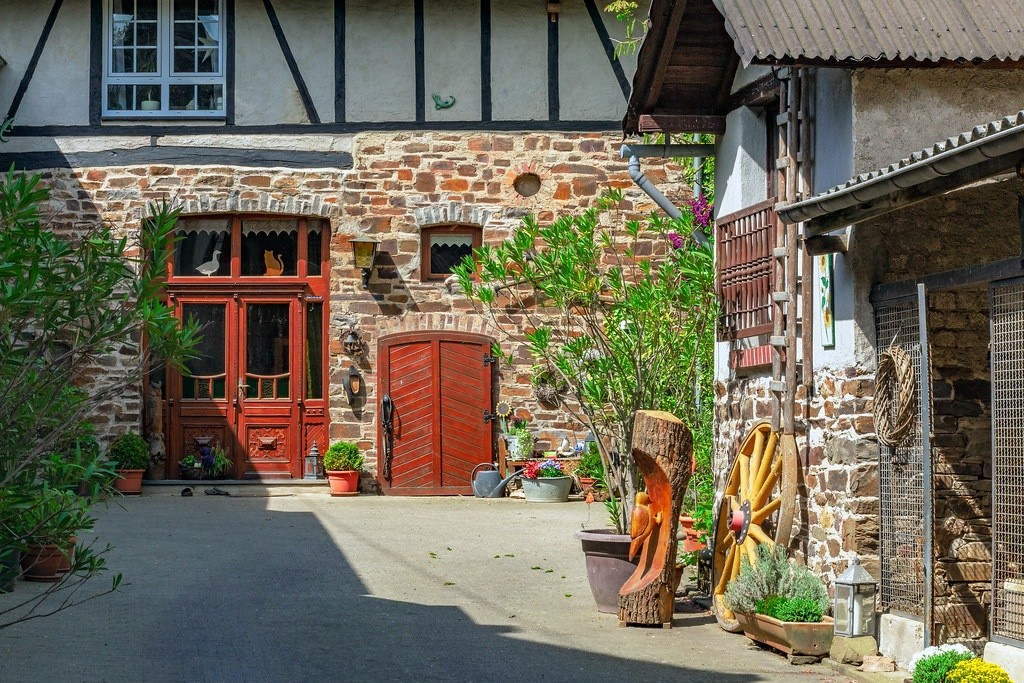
[
  {"xmin": 125, "ymin": 32, "xmax": 161, "ymax": 111},
  {"xmin": 449, "ymin": 184, "xmax": 739, "ymax": 614},
  {"xmin": 679, "ymin": 472, "xmax": 712, "ymax": 558},
  {"xmin": 15, "ymin": 488, "xmax": 72, "ymax": 581},
  {"xmin": 574, "ymin": 441, "xmax": 611, "ymax": 502},
  {"xmin": 912, "ymin": 650, "xmax": 975, "ymax": 683},
  {"xmin": 54, "ymin": 440, "xmax": 123, "ymax": 496},
  {"xmin": 106, "ymin": 432, "xmax": 149, "ymax": 495},
  {"xmin": 0, "ymin": 485, "xmax": 20, "ymax": 593},
  {"xmin": 38, "ymin": 488, "xmax": 82, "ymax": 572},
  {"xmin": 722, "ymin": 544, "xmax": 835, "ymax": 655},
  {"xmin": 324, "ymin": 441, "xmax": 363, "ymax": 496}
]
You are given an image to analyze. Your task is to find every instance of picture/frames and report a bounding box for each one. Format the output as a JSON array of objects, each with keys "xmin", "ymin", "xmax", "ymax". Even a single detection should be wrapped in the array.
[{"xmin": 818, "ymin": 234, "xmax": 834, "ymax": 346}]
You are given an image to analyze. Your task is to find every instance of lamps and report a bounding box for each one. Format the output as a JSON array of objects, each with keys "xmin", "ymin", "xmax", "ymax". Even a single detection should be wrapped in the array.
[
  {"xmin": 305, "ymin": 439, "xmax": 324, "ymax": 481},
  {"xmin": 347, "ymin": 231, "xmax": 381, "ymax": 288}
]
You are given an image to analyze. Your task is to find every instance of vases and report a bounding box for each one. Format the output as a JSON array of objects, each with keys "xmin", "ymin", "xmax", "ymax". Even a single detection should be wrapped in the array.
[
  {"xmin": 506, "ymin": 434, "xmax": 536, "ymax": 459},
  {"xmin": 519, "ymin": 473, "xmax": 572, "ymax": 502},
  {"xmin": 179, "ymin": 460, "xmax": 207, "ymax": 480}
]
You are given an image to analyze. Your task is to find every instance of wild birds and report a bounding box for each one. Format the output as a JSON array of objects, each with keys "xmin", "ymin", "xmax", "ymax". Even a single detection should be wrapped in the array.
[
  {"xmin": 194, "ymin": 249, "xmax": 221, "ymax": 277},
  {"xmin": 628, "ymin": 491, "xmax": 662, "ymax": 562}
]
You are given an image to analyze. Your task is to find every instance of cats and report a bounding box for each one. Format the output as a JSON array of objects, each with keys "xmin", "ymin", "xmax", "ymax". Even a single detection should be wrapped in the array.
[{"xmin": 262, "ymin": 249, "xmax": 284, "ymax": 276}]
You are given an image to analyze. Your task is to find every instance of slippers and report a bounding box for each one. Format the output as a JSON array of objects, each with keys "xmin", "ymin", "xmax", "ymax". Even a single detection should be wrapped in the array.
[
  {"xmin": 181, "ymin": 488, "xmax": 193, "ymax": 496},
  {"xmin": 204, "ymin": 488, "xmax": 228, "ymax": 495}
]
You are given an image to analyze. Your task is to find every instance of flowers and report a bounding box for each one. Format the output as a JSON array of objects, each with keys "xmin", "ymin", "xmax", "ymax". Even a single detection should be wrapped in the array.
[
  {"xmin": 909, "ymin": 644, "xmax": 975, "ymax": 673},
  {"xmin": 823, "ymin": 307, "xmax": 831, "ymax": 342},
  {"xmin": 495, "ymin": 399, "xmax": 512, "ymax": 434},
  {"xmin": 820, "ymin": 254, "xmax": 828, "ymax": 310},
  {"xmin": 508, "ymin": 416, "xmax": 532, "ymax": 435},
  {"xmin": 523, "ymin": 457, "xmax": 564, "ymax": 479},
  {"xmin": 945, "ymin": 657, "xmax": 1016, "ymax": 683},
  {"xmin": 181, "ymin": 442, "xmax": 231, "ymax": 475}
]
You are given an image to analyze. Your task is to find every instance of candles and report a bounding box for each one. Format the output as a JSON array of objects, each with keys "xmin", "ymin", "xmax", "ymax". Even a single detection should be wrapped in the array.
[{"xmin": 847, "ymin": 594, "xmax": 862, "ymax": 636}]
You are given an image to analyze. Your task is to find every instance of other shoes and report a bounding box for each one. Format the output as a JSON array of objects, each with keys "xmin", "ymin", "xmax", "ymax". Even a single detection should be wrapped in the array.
[
  {"xmin": 349, "ymin": 365, "xmax": 359, "ymax": 396},
  {"xmin": 342, "ymin": 377, "xmax": 354, "ymax": 405}
]
